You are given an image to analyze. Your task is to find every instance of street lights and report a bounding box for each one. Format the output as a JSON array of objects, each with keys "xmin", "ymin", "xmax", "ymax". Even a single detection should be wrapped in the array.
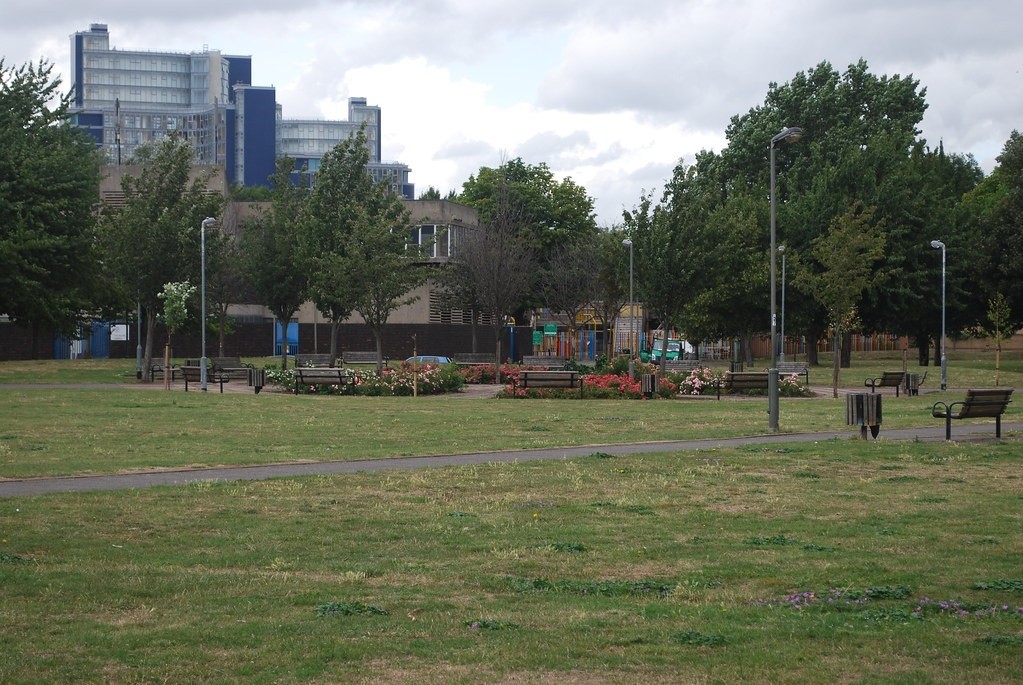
[
  {"xmin": 765, "ymin": 127, "xmax": 804, "ymax": 431},
  {"xmin": 931, "ymin": 240, "xmax": 948, "ymax": 391},
  {"xmin": 621, "ymin": 238, "xmax": 637, "ymax": 381},
  {"xmin": 777, "ymin": 245, "xmax": 785, "ymax": 362},
  {"xmin": 197, "ymin": 216, "xmax": 216, "ymax": 393}
]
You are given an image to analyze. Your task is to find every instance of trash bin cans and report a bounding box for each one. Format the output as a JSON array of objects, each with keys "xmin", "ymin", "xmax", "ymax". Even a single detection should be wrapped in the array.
[
  {"xmin": 246, "ymin": 368, "xmax": 265, "ymax": 386},
  {"xmin": 729, "ymin": 360, "xmax": 743, "ymax": 373},
  {"xmin": 641, "ymin": 374, "xmax": 660, "ymax": 393},
  {"xmin": 184, "ymin": 359, "xmax": 200, "ymax": 367},
  {"xmin": 845, "ymin": 391, "xmax": 883, "ymax": 425},
  {"xmin": 903, "ymin": 373, "xmax": 919, "ymax": 390}
]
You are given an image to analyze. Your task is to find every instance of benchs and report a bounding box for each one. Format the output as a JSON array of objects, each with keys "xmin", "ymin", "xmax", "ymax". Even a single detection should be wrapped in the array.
[
  {"xmin": 932, "ymin": 388, "xmax": 1015, "ymax": 441},
  {"xmin": 865, "ymin": 372, "xmax": 905, "ymax": 397},
  {"xmin": 293, "ymin": 368, "xmax": 359, "ymax": 396},
  {"xmin": 295, "ymin": 354, "xmax": 335, "ymax": 367},
  {"xmin": 338, "ymin": 351, "xmax": 389, "ymax": 368},
  {"xmin": 513, "ymin": 371, "xmax": 583, "ymax": 399},
  {"xmin": 150, "ymin": 358, "xmax": 181, "ymax": 382},
  {"xmin": 519, "ymin": 355, "xmax": 571, "ymax": 371},
  {"xmin": 664, "ymin": 360, "xmax": 702, "ymax": 373},
  {"xmin": 713, "ymin": 371, "xmax": 768, "ymax": 400},
  {"xmin": 776, "ymin": 362, "xmax": 809, "ymax": 385},
  {"xmin": 447, "ymin": 353, "xmax": 496, "ymax": 366},
  {"xmin": 180, "ymin": 366, "xmax": 230, "ymax": 393},
  {"xmin": 209, "ymin": 357, "xmax": 251, "ymax": 379}
]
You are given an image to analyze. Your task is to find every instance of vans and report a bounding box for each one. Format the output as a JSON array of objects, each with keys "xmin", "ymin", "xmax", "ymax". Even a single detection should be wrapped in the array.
[{"xmin": 639, "ymin": 336, "xmax": 686, "ymax": 364}]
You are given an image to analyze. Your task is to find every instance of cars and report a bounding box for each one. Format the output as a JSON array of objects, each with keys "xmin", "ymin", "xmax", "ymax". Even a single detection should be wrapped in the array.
[{"xmin": 404, "ymin": 356, "xmax": 454, "ymax": 363}]
